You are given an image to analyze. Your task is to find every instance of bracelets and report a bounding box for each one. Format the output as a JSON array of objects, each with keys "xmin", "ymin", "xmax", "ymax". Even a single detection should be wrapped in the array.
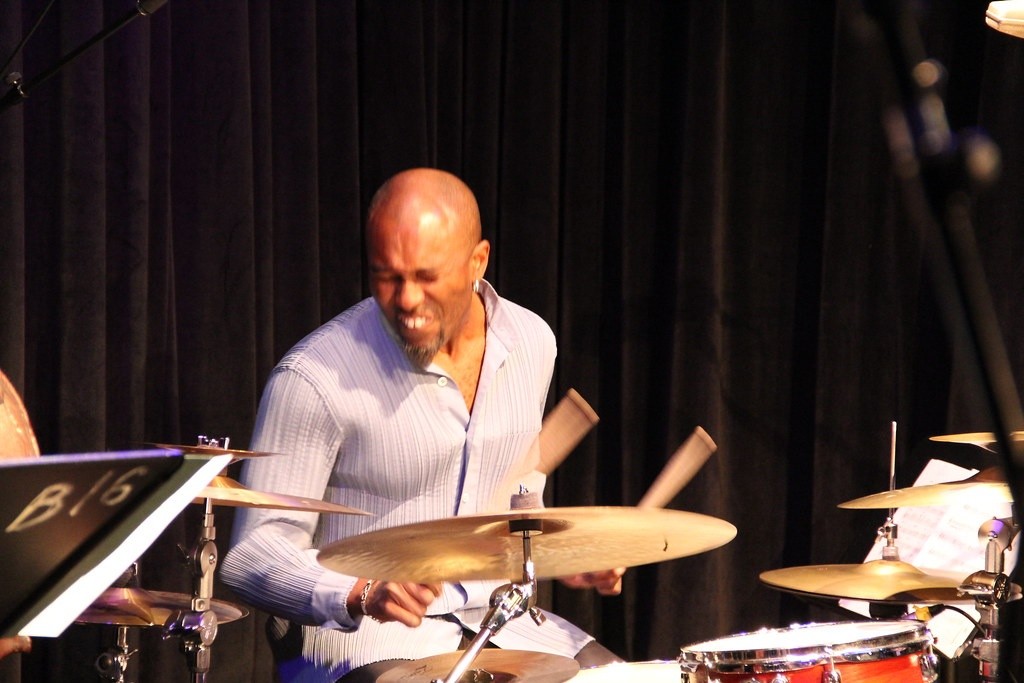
[{"xmin": 360, "ymin": 578, "xmax": 384, "ymax": 626}]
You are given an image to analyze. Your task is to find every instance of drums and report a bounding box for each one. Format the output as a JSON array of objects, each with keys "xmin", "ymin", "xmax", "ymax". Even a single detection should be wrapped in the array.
[{"xmin": 676, "ymin": 617, "xmax": 939, "ymax": 683}]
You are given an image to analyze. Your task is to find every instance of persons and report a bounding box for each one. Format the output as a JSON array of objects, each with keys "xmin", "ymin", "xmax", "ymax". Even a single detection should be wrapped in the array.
[{"xmin": 216, "ymin": 167, "xmax": 627, "ymax": 683}]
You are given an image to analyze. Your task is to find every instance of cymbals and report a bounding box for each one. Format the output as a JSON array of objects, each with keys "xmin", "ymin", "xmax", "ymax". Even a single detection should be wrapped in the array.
[
  {"xmin": 188, "ymin": 473, "xmax": 375, "ymax": 515},
  {"xmin": 757, "ymin": 554, "xmax": 1024, "ymax": 605},
  {"xmin": 153, "ymin": 439, "xmax": 285, "ymax": 459},
  {"xmin": 928, "ymin": 428, "xmax": 1024, "ymax": 447},
  {"xmin": 375, "ymin": 649, "xmax": 581, "ymax": 683},
  {"xmin": 315, "ymin": 504, "xmax": 739, "ymax": 585},
  {"xmin": 75, "ymin": 586, "xmax": 250, "ymax": 628},
  {"xmin": 835, "ymin": 462, "xmax": 1024, "ymax": 510}
]
